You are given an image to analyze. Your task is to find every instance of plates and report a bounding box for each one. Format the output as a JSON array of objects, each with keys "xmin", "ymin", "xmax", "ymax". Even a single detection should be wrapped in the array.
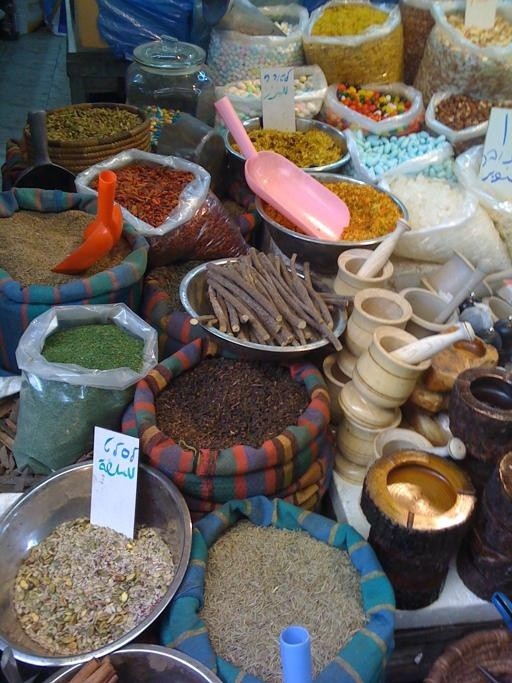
[{"xmin": 179, "ymin": 260, "xmax": 346, "ymax": 357}]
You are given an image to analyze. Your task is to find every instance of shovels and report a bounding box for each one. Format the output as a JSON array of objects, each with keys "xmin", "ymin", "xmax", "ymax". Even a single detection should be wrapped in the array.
[
  {"xmin": 12, "ymin": 110, "xmax": 77, "ymax": 194},
  {"xmin": 53, "ymin": 172, "xmax": 122, "ymax": 274},
  {"xmin": 215, "ymin": 96, "xmax": 350, "ymax": 243}
]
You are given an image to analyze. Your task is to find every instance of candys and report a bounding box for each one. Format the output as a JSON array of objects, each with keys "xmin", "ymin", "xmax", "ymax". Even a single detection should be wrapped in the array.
[
  {"xmin": 206, "ymin": 19, "xmax": 302, "ymax": 82},
  {"xmin": 386, "ymin": 173, "xmax": 512, "ymax": 273},
  {"xmin": 302, "ymin": 2, "xmax": 403, "ymax": 85},
  {"xmin": 138, "ymin": 105, "xmax": 182, "ymax": 146},
  {"xmin": 323, "ymin": 82, "xmax": 424, "ymax": 138},
  {"xmin": 214, "ymin": 71, "xmax": 322, "ymax": 138},
  {"xmin": 345, "ymin": 131, "xmax": 456, "ymax": 184}
]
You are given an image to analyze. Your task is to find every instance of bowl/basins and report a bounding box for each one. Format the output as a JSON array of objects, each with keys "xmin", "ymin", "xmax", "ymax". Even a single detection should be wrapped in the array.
[
  {"xmin": 39, "ymin": 642, "xmax": 224, "ymax": 683},
  {"xmin": 254, "ymin": 171, "xmax": 410, "ymax": 276},
  {"xmin": 0, "ymin": 462, "xmax": 194, "ymax": 666},
  {"xmin": 224, "ymin": 117, "xmax": 352, "ymax": 170}
]
